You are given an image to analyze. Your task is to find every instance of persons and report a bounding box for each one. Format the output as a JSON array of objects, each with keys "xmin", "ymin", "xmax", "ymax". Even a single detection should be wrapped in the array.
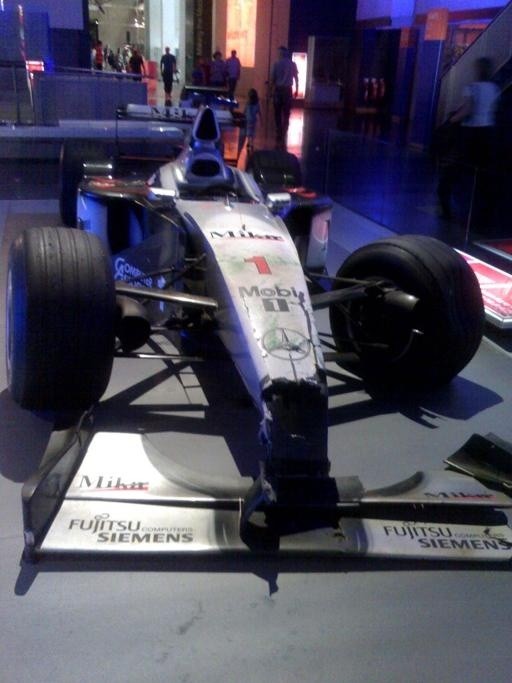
[
  {"xmin": 160, "ymin": 46, "xmax": 177, "ymax": 104},
  {"xmin": 224, "ymin": 49, "xmax": 241, "ymax": 100},
  {"xmin": 91, "ymin": 40, "xmax": 132, "ymax": 72},
  {"xmin": 209, "ymin": 50, "xmax": 225, "ymax": 86},
  {"xmin": 242, "ymin": 85, "xmax": 263, "ymax": 147},
  {"xmin": 435, "ymin": 56, "xmax": 507, "ymax": 225},
  {"xmin": 128, "ymin": 50, "xmax": 147, "ymax": 81},
  {"xmin": 266, "ymin": 44, "xmax": 298, "ymax": 131}
]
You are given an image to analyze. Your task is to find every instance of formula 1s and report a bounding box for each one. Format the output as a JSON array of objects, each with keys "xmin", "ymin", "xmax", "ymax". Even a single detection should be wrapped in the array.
[{"xmin": 3, "ymin": 100, "xmax": 512, "ymax": 565}]
